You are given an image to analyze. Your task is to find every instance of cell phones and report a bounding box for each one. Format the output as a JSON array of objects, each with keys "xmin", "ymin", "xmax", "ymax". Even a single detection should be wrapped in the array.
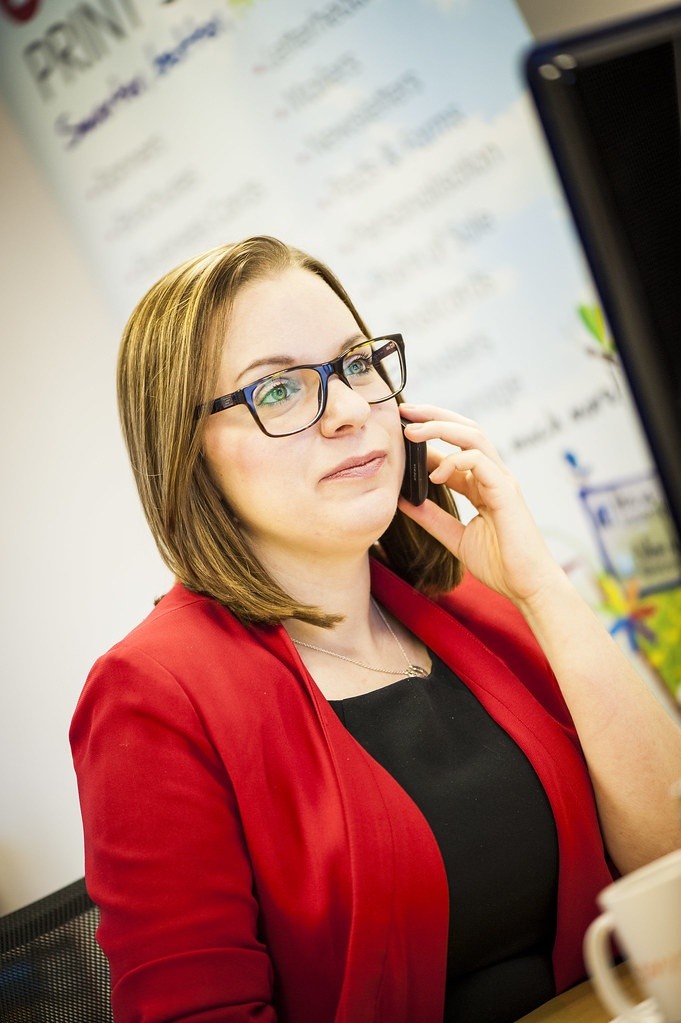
[{"xmin": 401, "ymin": 416, "xmax": 428, "ymax": 507}]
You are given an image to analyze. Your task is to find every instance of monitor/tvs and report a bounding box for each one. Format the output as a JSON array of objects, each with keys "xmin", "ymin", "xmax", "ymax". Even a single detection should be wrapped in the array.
[{"xmin": 520, "ymin": 0, "xmax": 681, "ymax": 527}]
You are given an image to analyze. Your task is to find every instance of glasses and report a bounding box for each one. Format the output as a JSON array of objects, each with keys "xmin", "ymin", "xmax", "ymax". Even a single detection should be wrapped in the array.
[{"xmin": 191, "ymin": 333, "xmax": 405, "ymax": 435}]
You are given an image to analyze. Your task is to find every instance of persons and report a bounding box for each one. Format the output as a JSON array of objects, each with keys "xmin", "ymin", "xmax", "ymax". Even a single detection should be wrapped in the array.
[{"xmin": 67, "ymin": 233, "xmax": 681, "ymax": 1022}]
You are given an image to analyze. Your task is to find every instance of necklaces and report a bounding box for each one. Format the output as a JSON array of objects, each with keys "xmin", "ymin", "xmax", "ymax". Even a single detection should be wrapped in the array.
[{"xmin": 289, "ymin": 595, "xmax": 429, "ymax": 679}]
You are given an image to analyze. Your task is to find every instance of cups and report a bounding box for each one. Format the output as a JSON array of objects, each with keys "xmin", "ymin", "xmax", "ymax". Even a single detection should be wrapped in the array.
[{"xmin": 583, "ymin": 848, "xmax": 681, "ymax": 1023}]
